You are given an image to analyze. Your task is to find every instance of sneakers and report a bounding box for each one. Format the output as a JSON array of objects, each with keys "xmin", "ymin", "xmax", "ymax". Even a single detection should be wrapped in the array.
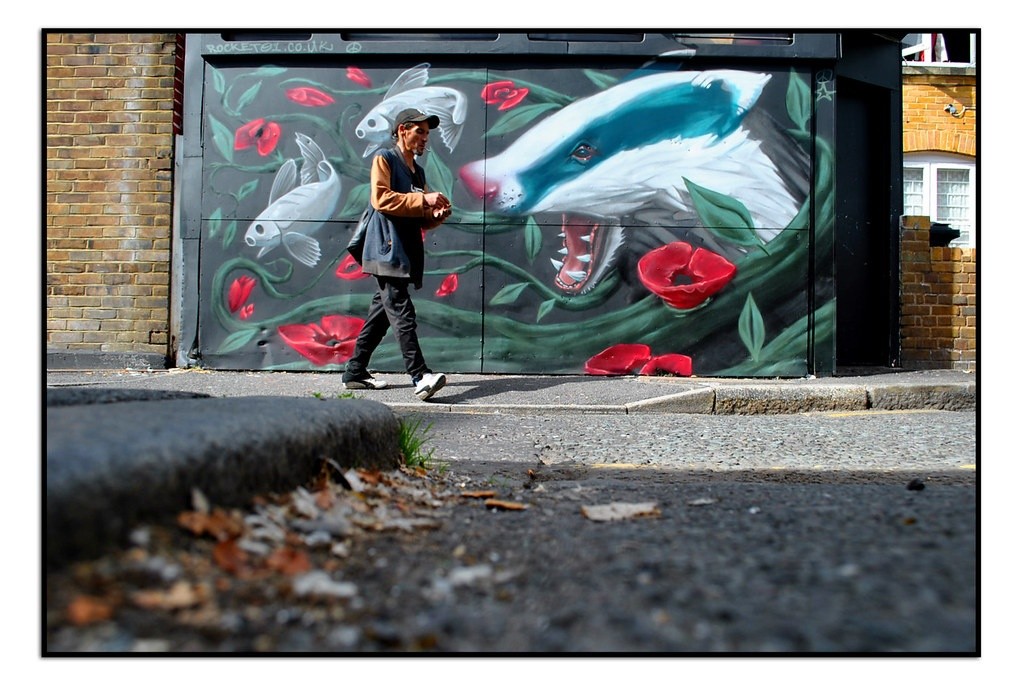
[
  {"xmin": 414, "ymin": 371, "xmax": 446, "ymax": 400},
  {"xmin": 343, "ymin": 378, "xmax": 388, "ymax": 389}
]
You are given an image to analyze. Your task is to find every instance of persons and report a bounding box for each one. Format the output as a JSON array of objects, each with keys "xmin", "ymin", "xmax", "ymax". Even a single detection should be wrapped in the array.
[{"xmin": 342, "ymin": 107, "xmax": 453, "ymax": 402}]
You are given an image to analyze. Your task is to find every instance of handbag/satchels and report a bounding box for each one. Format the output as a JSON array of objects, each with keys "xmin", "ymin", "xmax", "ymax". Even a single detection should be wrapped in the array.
[{"xmin": 347, "ymin": 209, "xmax": 372, "ymax": 267}]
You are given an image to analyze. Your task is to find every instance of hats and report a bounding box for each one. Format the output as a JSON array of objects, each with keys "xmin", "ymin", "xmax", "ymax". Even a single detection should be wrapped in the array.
[{"xmin": 392, "ymin": 108, "xmax": 440, "ymax": 137}]
